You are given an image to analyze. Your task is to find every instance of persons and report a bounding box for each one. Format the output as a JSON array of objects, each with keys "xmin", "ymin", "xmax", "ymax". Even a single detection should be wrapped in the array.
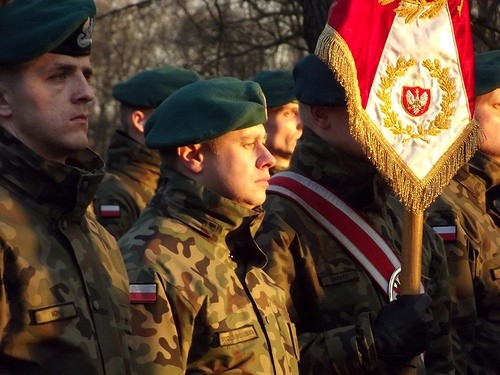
[
  {"xmin": 425, "ymin": 50, "xmax": 500, "ymax": 375},
  {"xmin": 120, "ymin": 77, "xmax": 300, "ymax": 375},
  {"xmin": 254, "ymin": 57, "xmax": 456, "ymax": 375},
  {"xmin": 251, "ymin": 72, "xmax": 303, "ymax": 178},
  {"xmin": 93, "ymin": 66, "xmax": 203, "ymax": 240},
  {"xmin": 0, "ymin": 1, "xmax": 135, "ymax": 375}
]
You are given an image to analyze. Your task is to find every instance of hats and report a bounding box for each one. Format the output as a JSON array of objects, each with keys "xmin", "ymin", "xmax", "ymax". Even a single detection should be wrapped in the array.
[
  {"xmin": 0, "ymin": 1, "xmax": 97, "ymax": 65},
  {"xmin": 113, "ymin": 66, "xmax": 202, "ymax": 111},
  {"xmin": 142, "ymin": 76, "xmax": 267, "ymax": 156},
  {"xmin": 474, "ymin": 50, "xmax": 500, "ymax": 95},
  {"xmin": 293, "ymin": 54, "xmax": 348, "ymax": 108},
  {"xmin": 253, "ymin": 70, "xmax": 298, "ymax": 108}
]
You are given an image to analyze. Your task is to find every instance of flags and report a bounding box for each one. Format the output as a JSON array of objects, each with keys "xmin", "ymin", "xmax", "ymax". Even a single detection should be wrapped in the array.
[{"xmin": 314, "ymin": 1, "xmax": 488, "ymax": 216}]
